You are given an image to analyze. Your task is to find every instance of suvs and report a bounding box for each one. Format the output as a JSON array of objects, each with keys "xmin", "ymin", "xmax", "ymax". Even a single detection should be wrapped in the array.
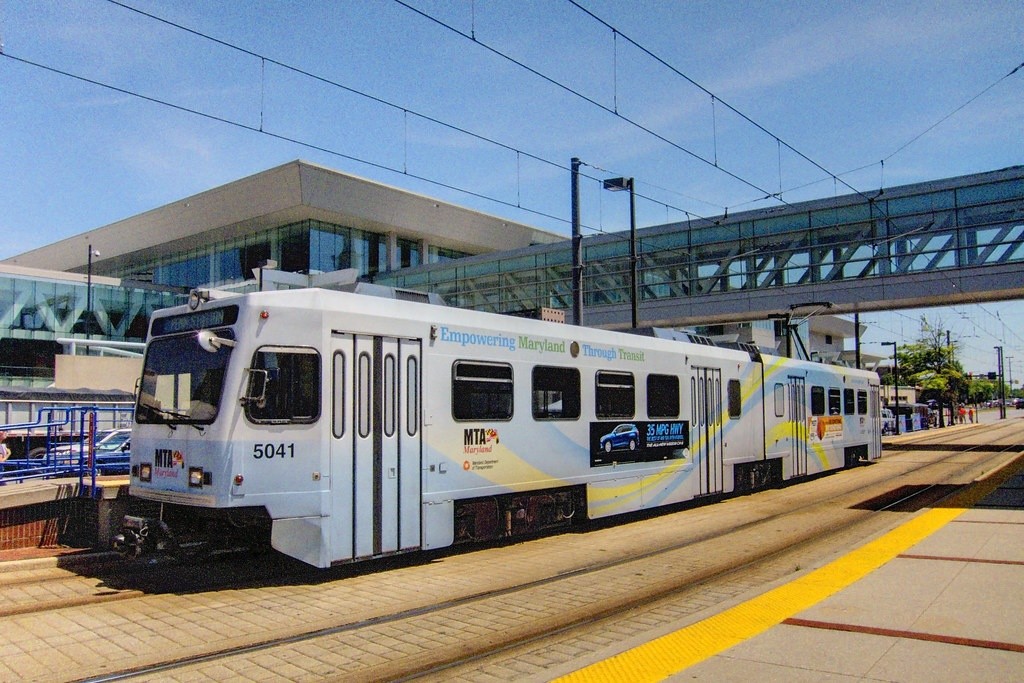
[
  {"xmin": 880, "ymin": 407, "xmax": 896, "ymax": 434},
  {"xmin": 599, "ymin": 423, "xmax": 640, "ymax": 453},
  {"xmin": 43, "ymin": 427, "xmax": 114, "ymax": 471}
]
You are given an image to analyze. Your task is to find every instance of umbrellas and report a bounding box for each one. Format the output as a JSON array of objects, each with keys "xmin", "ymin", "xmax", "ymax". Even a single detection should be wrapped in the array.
[{"xmin": 927, "ymin": 399, "xmax": 937, "ymax": 403}]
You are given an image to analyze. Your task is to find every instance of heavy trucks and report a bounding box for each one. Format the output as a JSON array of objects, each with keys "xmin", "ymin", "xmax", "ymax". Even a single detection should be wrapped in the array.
[{"xmin": 0, "ymin": 384, "xmax": 134, "ymax": 475}]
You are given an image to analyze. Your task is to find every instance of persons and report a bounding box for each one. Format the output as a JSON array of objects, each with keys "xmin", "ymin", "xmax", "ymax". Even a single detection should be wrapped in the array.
[
  {"xmin": 0, "ymin": 430, "xmax": 12, "ymax": 486},
  {"xmin": 958, "ymin": 406, "xmax": 967, "ymax": 424},
  {"xmin": 980, "ymin": 402, "xmax": 983, "ymax": 408},
  {"xmin": 881, "ymin": 405, "xmax": 887, "ymax": 418},
  {"xmin": 968, "ymin": 406, "xmax": 974, "ymax": 423}
]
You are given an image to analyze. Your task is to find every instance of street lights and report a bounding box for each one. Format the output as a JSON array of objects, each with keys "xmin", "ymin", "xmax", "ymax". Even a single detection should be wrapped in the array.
[
  {"xmin": 603, "ymin": 175, "xmax": 637, "ymax": 330},
  {"xmin": 1006, "ymin": 356, "xmax": 1014, "ymax": 396},
  {"xmin": 880, "ymin": 342, "xmax": 901, "ymax": 434}
]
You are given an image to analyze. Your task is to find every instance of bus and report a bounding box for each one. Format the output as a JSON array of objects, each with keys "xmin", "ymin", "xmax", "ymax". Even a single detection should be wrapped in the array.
[
  {"xmin": 112, "ymin": 264, "xmax": 882, "ymax": 568},
  {"xmin": 885, "ymin": 402, "xmax": 929, "ymax": 430}
]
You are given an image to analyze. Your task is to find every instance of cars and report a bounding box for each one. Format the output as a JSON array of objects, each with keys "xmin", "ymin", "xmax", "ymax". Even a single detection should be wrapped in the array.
[
  {"xmin": 56, "ymin": 435, "xmax": 132, "ymax": 476},
  {"xmin": 986, "ymin": 398, "xmax": 1024, "ymax": 409}
]
